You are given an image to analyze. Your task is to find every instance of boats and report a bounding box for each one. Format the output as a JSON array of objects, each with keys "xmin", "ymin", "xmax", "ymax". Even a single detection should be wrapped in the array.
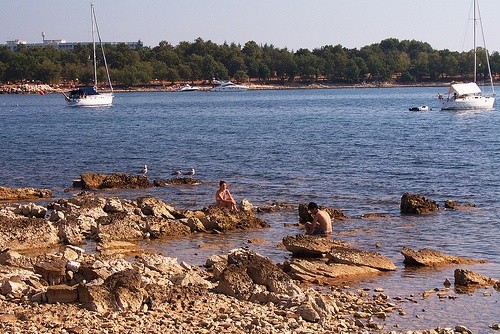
[
  {"xmin": 177, "ymin": 84, "xmax": 199, "ymax": 93},
  {"xmin": 210, "ymin": 80, "xmax": 249, "ymax": 92}
]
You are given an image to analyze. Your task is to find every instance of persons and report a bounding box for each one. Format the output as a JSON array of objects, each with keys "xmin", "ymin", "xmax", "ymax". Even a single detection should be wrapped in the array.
[
  {"xmin": 216, "ymin": 180, "xmax": 240, "ymax": 213},
  {"xmin": 304, "ymin": 202, "xmax": 332, "ymax": 236}
]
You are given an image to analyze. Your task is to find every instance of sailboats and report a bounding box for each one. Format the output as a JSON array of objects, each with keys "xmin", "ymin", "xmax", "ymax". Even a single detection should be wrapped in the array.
[
  {"xmin": 61, "ymin": 2, "xmax": 116, "ymax": 107},
  {"xmin": 438, "ymin": 0, "xmax": 497, "ymax": 111}
]
